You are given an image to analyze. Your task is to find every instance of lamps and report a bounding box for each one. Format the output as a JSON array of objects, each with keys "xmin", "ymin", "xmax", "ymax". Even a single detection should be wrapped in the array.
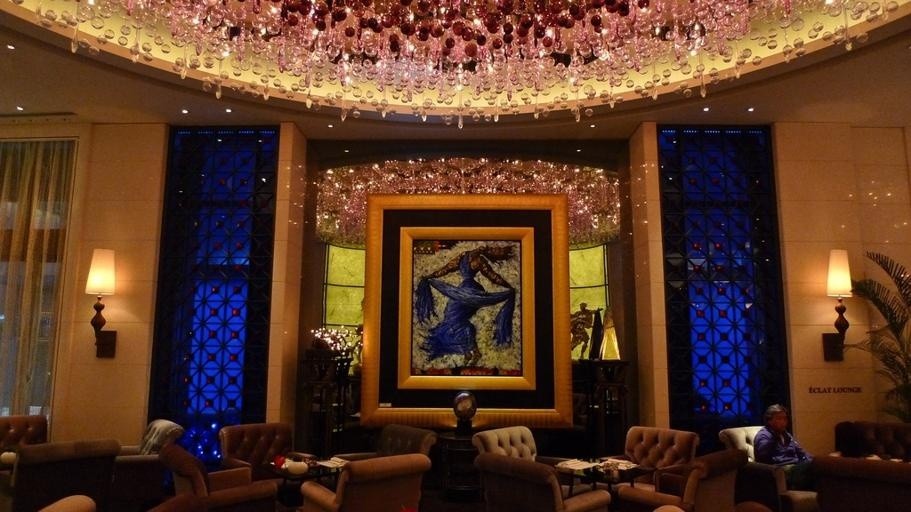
[
  {"xmin": 308, "ymin": 157, "xmax": 621, "ymax": 247},
  {"xmin": 823, "ymin": 246, "xmax": 859, "ymax": 364},
  {"xmin": 13, "ymin": 0, "xmax": 905, "ymax": 130},
  {"xmin": 81, "ymin": 245, "xmax": 122, "ymax": 361}
]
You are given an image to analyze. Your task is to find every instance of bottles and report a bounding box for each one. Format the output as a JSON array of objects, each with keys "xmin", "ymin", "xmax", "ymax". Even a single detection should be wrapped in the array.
[{"xmin": 288, "ymin": 457, "xmax": 309, "ymax": 475}]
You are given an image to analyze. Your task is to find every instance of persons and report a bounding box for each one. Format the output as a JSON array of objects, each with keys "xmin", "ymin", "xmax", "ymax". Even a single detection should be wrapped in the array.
[
  {"xmin": 569, "ymin": 303, "xmax": 603, "ymax": 360},
  {"xmin": 753, "ymin": 403, "xmax": 820, "ymax": 491},
  {"xmin": 423, "ymin": 244, "xmax": 514, "ymax": 368}
]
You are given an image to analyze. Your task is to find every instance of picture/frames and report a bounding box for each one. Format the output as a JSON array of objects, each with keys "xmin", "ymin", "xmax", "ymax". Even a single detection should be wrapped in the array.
[{"xmin": 396, "ymin": 224, "xmax": 538, "ymax": 394}]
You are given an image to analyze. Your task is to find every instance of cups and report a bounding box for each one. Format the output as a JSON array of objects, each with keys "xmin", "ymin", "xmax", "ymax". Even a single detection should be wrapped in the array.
[{"xmin": 601, "ymin": 461, "xmax": 620, "ymax": 482}]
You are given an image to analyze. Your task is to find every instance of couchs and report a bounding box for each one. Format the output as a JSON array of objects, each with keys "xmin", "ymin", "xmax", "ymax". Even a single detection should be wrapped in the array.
[{"xmin": 1, "ymin": 411, "xmax": 884, "ymax": 512}]
